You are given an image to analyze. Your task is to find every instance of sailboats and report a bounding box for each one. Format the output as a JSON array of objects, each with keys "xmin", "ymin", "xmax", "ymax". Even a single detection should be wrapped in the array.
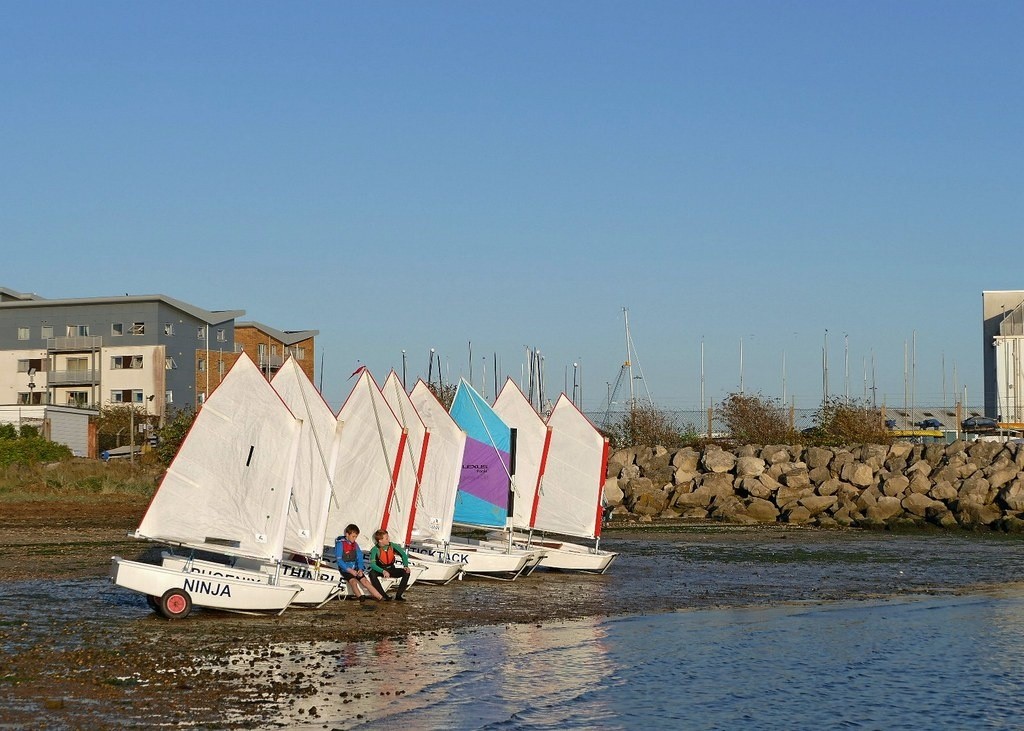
[
  {"xmin": 110, "ymin": 350, "xmax": 304, "ymax": 612},
  {"xmin": 164, "ymin": 349, "xmax": 620, "ymax": 606}
]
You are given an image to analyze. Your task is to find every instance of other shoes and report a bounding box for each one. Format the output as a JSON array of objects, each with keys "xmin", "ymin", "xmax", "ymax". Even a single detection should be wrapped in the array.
[
  {"xmin": 384, "ymin": 594, "xmax": 392, "ymax": 601},
  {"xmin": 359, "ymin": 594, "xmax": 365, "ymax": 602},
  {"xmin": 394, "ymin": 596, "xmax": 407, "ymax": 601},
  {"xmin": 379, "ymin": 596, "xmax": 390, "ymax": 604}
]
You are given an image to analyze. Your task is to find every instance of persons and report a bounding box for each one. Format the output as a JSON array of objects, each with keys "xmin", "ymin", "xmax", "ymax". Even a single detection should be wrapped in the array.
[
  {"xmin": 336, "ymin": 523, "xmax": 383, "ymax": 602},
  {"xmin": 369, "ymin": 529, "xmax": 411, "ymax": 602}
]
